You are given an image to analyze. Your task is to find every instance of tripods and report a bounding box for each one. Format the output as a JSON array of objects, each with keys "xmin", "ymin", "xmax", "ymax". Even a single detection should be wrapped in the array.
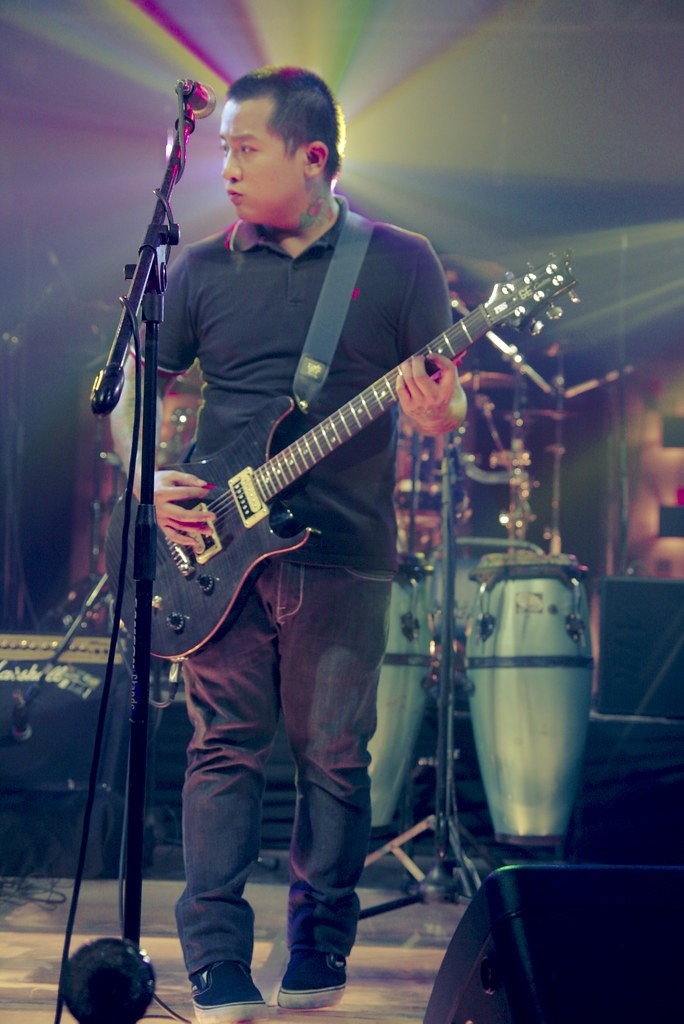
[{"xmin": 357, "ymin": 417, "xmax": 505, "ymax": 918}]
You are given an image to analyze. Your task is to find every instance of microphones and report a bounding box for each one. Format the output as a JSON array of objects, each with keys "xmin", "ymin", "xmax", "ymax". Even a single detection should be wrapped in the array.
[
  {"xmin": 12, "ymin": 694, "xmax": 32, "ymax": 741},
  {"xmin": 175, "ymin": 79, "xmax": 216, "ymax": 118}
]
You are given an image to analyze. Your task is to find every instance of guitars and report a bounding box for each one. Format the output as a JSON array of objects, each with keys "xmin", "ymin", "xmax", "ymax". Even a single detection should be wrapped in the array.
[{"xmin": 101, "ymin": 245, "xmax": 584, "ymax": 664}]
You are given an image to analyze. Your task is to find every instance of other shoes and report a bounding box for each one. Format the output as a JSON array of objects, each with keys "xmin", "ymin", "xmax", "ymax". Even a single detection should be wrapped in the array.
[
  {"xmin": 190, "ymin": 959, "xmax": 267, "ymax": 1024},
  {"xmin": 273, "ymin": 949, "xmax": 349, "ymax": 1009}
]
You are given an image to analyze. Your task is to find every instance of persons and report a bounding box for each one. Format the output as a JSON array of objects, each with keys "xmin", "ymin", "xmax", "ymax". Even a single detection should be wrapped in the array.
[{"xmin": 110, "ymin": 65, "xmax": 468, "ymax": 1024}]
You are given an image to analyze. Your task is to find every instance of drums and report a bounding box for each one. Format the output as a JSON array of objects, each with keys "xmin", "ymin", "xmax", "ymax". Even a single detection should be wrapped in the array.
[
  {"xmin": 366, "ymin": 555, "xmax": 431, "ymax": 830},
  {"xmin": 460, "ymin": 549, "xmax": 596, "ymax": 851},
  {"xmin": 394, "ymin": 479, "xmax": 442, "ymax": 527},
  {"xmin": 431, "ymin": 537, "xmax": 544, "ymax": 644}
]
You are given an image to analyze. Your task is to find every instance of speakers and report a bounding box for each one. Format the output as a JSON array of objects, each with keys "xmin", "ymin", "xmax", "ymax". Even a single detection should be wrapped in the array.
[
  {"xmin": 587, "ymin": 577, "xmax": 684, "ymax": 721},
  {"xmin": 0, "ymin": 631, "xmax": 131, "ymax": 795},
  {"xmin": 424, "ymin": 864, "xmax": 684, "ymax": 1024},
  {"xmin": 566, "ymin": 720, "xmax": 684, "ymax": 865}
]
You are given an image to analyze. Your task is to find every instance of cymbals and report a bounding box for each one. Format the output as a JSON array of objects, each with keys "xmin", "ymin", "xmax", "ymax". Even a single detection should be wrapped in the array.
[
  {"xmin": 458, "ymin": 367, "xmax": 517, "ymax": 393},
  {"xmin": 498, "ymin": 404, "xmax": 571, "ymax": 423}
]
